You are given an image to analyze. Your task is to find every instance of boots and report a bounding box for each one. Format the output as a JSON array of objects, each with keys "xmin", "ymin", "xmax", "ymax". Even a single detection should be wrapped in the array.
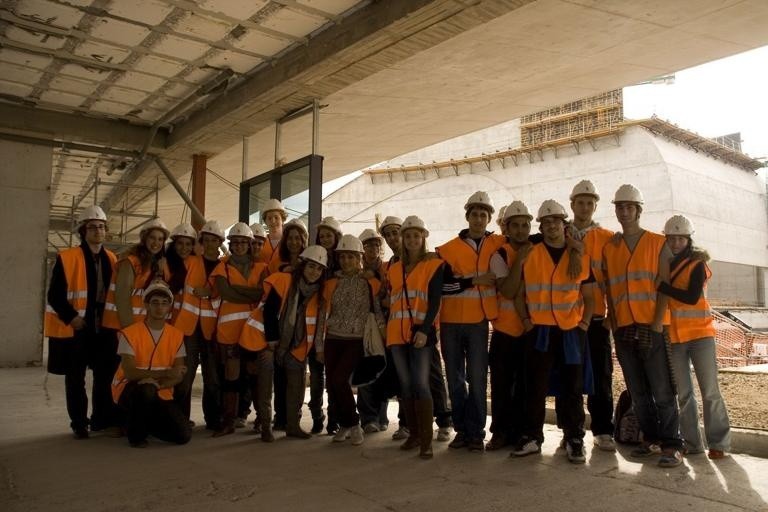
[
  {"xmin": 252, "ymin": 394, "xmax": 262, "ymax": 430},
  {"xmin": 212, "ymin": 391, "xmax": 240, "ymax": 438},
  {"xmin": 401, "ymin": 400, "xmax": 419, "ymax": 451},
  {"xmin": 414, "ymin": 398, "xmax": 434, "ymax": 458}
]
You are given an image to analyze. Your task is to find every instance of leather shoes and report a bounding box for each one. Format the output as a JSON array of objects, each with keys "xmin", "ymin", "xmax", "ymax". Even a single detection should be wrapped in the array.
[{"xmin": 69, "ymin": 422, "xmax": 89, "ymax": 439}]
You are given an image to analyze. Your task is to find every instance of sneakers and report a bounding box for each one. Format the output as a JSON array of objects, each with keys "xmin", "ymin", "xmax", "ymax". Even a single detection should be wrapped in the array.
[
  {"xmin": 261, "ymin": 416, "xmax": 340, "ymax": 443},
  {"xmin": 332, "ymin": 421, "xmax": 388, "ymax": 446},
  {"xmin": 630, "ymin": 443, "xmax": 661, "ymax": 457},
  {"xmin": 449, "ymin": 431, "xmax": 526, "ymax": 452},
  {"xmin": 593, "ymin": 434, "xmax": 616, "ymax": 450},
  {"xmin": 205, "ymin": 416, "xmax": 247, "ymax": 431},
  {"xmin": 559, "ymin": 437, "xmax": 585, "ymax": 463},
  {"xmin": 658, "ymin": 446, "xmax": 688, "ymax": 467},
  {"xmin": 391, "ymin": 426, "xmax": 410, "ymax": 440},
  {"xmin": 509, "ymin": 436, "xmax": 541, "ymax": 458},
  {"xmin": 708, "ymin": 450, "xmax": 722, "ymax": 459},
  {"xmin": 436, "ymin": 427, "xmax": 451, "ymax": 442}
]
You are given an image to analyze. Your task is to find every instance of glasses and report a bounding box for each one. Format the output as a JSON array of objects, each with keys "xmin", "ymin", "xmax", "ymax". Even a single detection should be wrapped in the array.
[
  {"xmin": 85, "ymin": 224, "xmax": 104, "ymax": 230},
  {"xmin": 230, "ymin": 239, "xmax": 248, "ymax": 246}
]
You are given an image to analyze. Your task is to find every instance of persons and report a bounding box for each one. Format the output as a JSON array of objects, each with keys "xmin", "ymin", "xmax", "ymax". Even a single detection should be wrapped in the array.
[
  {"xmin": 601, "ymin": 182, "xmax": 685, "ymax": 467},
  {"xmin": 435, "ymin": 191, "xmax": 584, "ymax": 455},
  {"xmin": 495, "ymin": 205, "xmax": 508, "ymax": 235},
  {"xmin": 509, "ymin": 198, "xmax": 597, "ymax": 464},
  {"xmin": 485, "ymin": 201, "xmax": 582, "ymax": 453},
  {"xmin": 563, "ymin": 179, "xmax": 616, "ymax": 451},
  {"xmin": 610, "ymin": 213, "xmax": 733, "ymax": 460},
  {"xmin": 40, "ymin": 197, "xmax": 446, "ymax": 457}
]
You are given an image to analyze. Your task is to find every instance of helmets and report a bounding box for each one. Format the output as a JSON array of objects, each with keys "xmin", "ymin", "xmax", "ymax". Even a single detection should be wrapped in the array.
[
  {"xmin": 663, "ymin": 215, "xmax": 694, "ymax": 235},
  {"xmin": 171, "ymin": 220, "xmax": 267, "ymax": 240},
  {"xmin": 463, "ymin": 191, "xmax": 568, "ymax": 222},
  {"xmin": 569, "ymin": 180, "xmax": 600, "ymax": 200},
  {"xmin": 141, "ymin": 280, "xmax": 174, "ymax": 305},
  {"xmin": 282, "ymin": 215, "xmax": 403, "ymax": 269},
  {"xmin": 612, "ymin": 184, "xmax": 644, "ymax": 204},
  {"xmin": 261, "ymin": 198, "xmax": 286, "ymax": 223},
  {"xmin": 139, "ymin": 218, "xmax": 171, "ymax": 240},
  {"xmin": 78, "ymin": 205, "xmax": 106, "ymax": 223},
  {"xmin": 396, "ymin": 215, "xmax": 430, "ymax": 236}
]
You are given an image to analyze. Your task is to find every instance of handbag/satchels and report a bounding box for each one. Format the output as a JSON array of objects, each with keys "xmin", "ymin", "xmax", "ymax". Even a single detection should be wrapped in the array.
[
  {"xmin": 410, "ymin": 322, "xmax": 437, "ymax": 348},
  {"xmin": 613, "ymin": 390, "xmax": 644, "ymax": 444}
]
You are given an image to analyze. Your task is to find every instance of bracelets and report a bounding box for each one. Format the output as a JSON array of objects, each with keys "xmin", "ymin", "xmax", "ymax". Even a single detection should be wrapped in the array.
[
  {"xmin": 581, "ymin": 319, "xmax": 590, "ymax": 327},
  {"xmin": 520, "ymin": 317, "xmax": 529, "ymax": 324}
]
